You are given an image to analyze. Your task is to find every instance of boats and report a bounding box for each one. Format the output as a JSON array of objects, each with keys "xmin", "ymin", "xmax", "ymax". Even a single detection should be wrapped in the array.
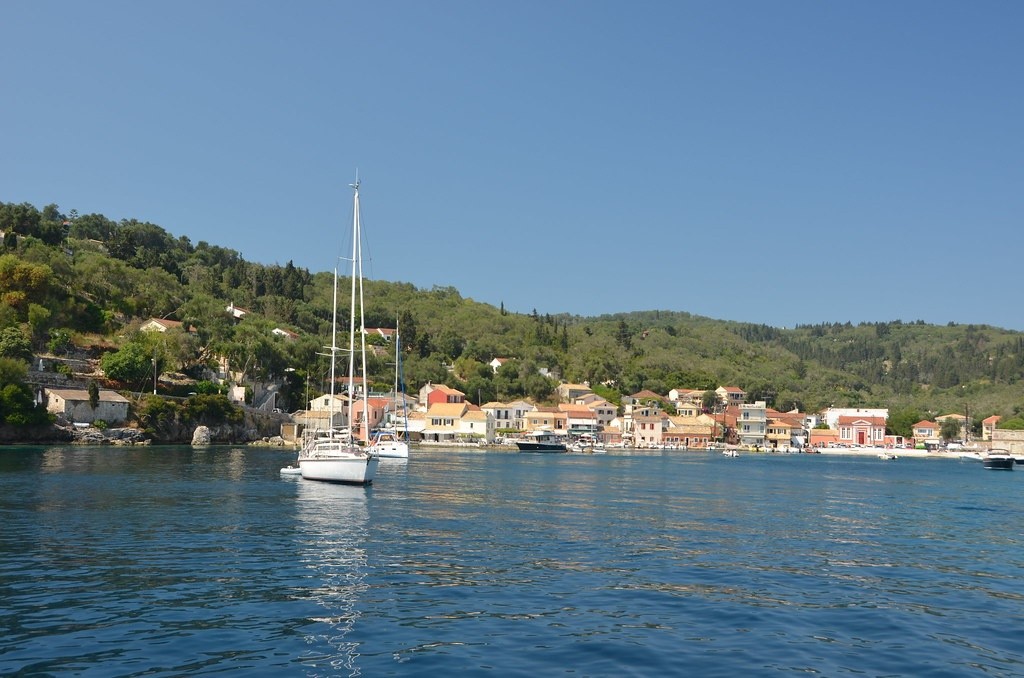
[
  {"xmin": 515, "ymin": 428, "xmax": 569, "ymax": 453},
  {"xmin": 983, "ymin": 447, "xmax": 1014, "ymax": 471},
  {"xmin": 878, "ymin": 452, "xmax": 898, "ymax": 459},
  {"xmin": 805, "ymin": 448, "xmax": 821, "ymax": 454}
]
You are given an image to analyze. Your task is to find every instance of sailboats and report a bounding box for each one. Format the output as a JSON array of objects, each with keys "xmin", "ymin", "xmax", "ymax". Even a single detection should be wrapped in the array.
[
  {"xmin": 367, "ymin": 305, "xmax": 411, "ymax": 459},
  {"xmin": 298, "ymin": 164, "xmax": 383, "ymax": 484}
]
